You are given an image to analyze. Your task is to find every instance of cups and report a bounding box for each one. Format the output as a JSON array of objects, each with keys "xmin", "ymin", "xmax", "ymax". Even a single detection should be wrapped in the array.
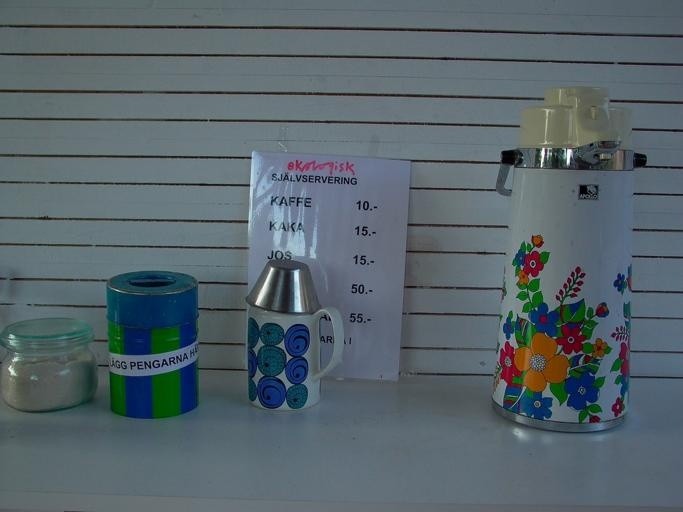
[
  {"xmin": 491, "ymin": 87, "xmax": 646, "ymax": 432},
  {"xmin": 248, "ymin": 309, "xmax": 344, "ymax": 411}
]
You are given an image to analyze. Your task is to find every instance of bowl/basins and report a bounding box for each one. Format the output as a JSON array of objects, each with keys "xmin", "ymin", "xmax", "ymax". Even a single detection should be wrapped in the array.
[{"xmin": 244, "ymin": 260, "xmax": 322, "ymax": 313}]
